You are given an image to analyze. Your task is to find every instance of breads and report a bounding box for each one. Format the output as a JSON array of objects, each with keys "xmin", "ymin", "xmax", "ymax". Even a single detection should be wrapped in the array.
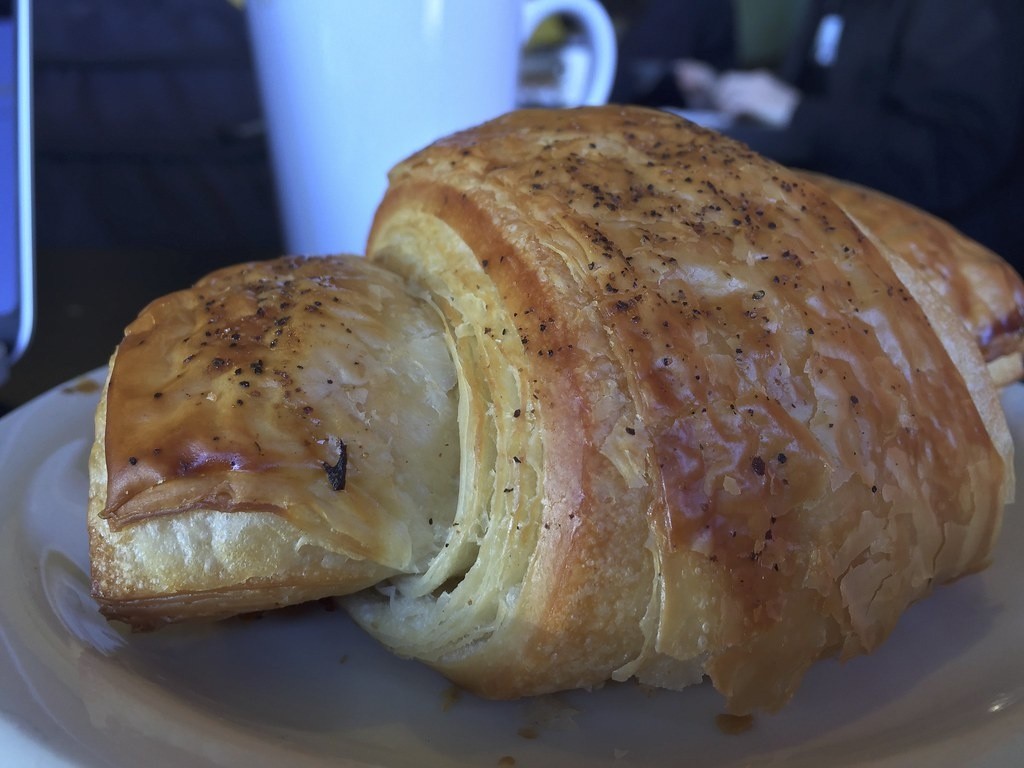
[{"xmin": 84, "ymin": 104, "xmax": 1024, "ymax": 727}]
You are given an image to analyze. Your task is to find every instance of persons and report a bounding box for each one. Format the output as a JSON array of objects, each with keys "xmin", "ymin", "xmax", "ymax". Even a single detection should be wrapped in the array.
[
  {"xmin": 674, "ymin": 0, "xmax": 1024, "ymax": 281},
  {"xmin": 601, "ymin": 1, "xmax": 741, "ymax": 109}
]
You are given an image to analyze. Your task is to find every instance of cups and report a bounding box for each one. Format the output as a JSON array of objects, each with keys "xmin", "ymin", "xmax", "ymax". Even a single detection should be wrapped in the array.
[{"xmin": 244, "ymin": 0, "xmax": 619, "ymax": 256}]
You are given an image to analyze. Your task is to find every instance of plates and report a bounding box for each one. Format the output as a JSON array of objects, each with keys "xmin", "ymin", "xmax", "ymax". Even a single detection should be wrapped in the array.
[{"xmin": 0, "ymin": 362, "xmax": 1024, "ymax": 768}]
